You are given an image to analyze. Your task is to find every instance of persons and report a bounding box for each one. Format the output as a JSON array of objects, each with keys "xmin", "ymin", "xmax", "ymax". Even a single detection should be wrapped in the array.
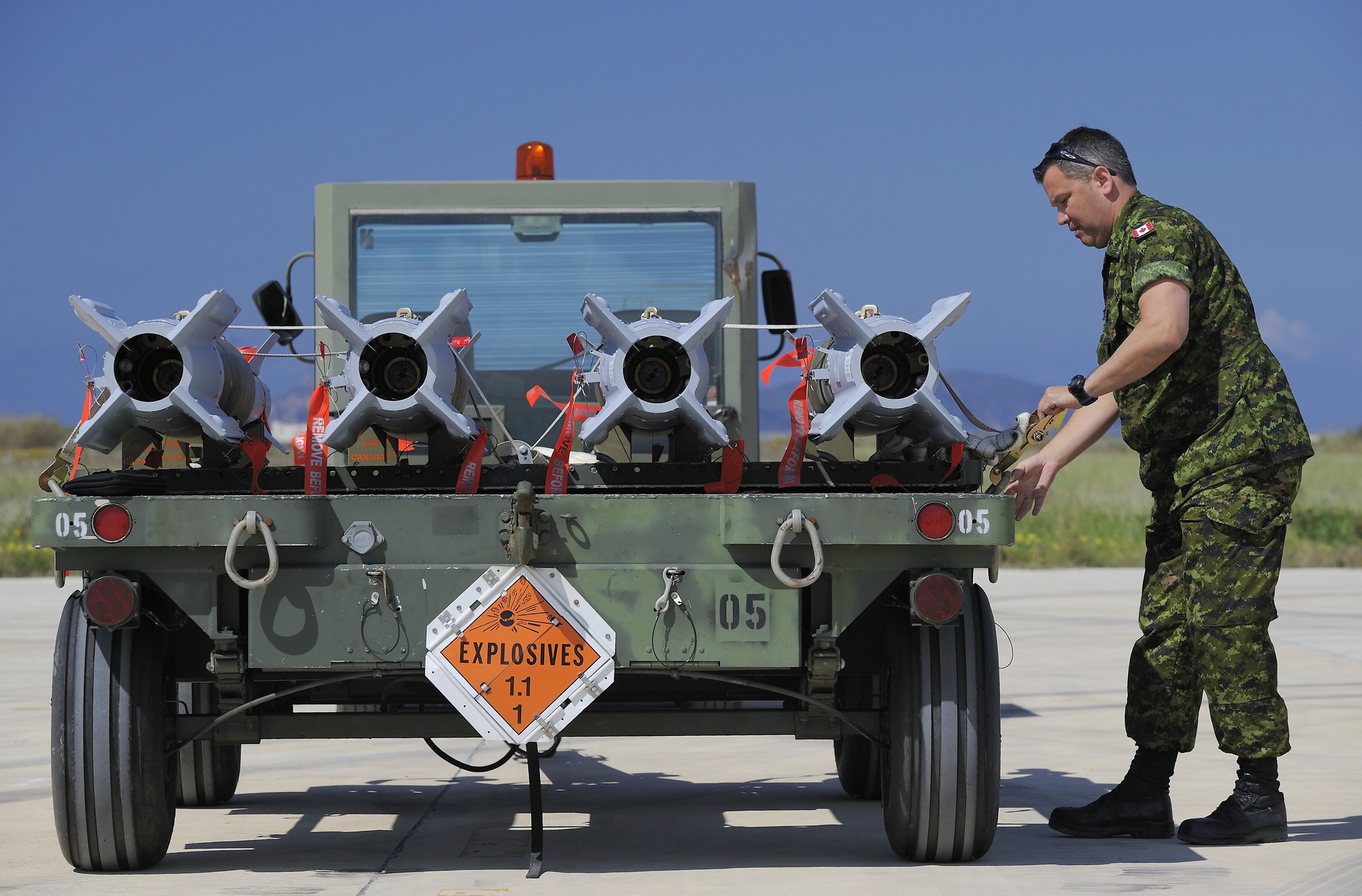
[{"xmin": 1003, "ymin": 127, "xmax": 1315, "ymax": 846}]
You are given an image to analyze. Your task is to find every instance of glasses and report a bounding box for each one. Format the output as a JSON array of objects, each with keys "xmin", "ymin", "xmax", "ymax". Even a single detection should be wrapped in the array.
[{"xmin": 1045, "ymin": 143, "xmax": 1117, "ymax": 176}]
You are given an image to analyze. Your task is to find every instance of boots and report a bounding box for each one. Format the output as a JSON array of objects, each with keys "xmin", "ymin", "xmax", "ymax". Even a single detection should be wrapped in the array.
[
  {"xmin": 1049, "ymin": 740, "xmax": 1175, "ymax": 839},
  {"xmin": 1178, "ymin": 755, "xmax": 1289, "ymax": 844}
]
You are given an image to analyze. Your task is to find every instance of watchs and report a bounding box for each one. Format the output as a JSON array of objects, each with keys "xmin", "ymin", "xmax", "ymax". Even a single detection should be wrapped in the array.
[{"xmin": 1068, "ymin": 375, "xmax": 1098, "ymax": 406}]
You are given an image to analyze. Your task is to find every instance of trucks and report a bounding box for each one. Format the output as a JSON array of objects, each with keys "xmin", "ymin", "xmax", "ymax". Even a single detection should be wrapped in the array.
[{"xmin": 31, "ymin": 139, "xmax": 1026, "ymax": 872}]
